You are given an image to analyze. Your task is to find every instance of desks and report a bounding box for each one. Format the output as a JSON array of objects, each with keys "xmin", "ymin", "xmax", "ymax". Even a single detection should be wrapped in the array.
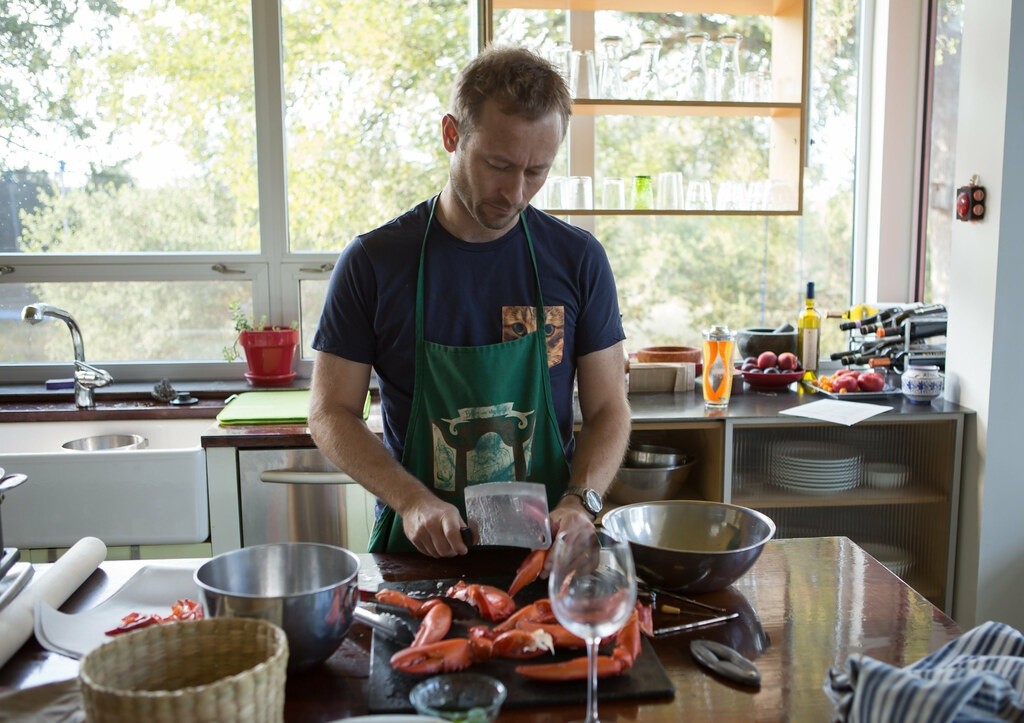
[{"xmin": 1, "ymin": 536, "xmax": 963, "ymax": 723}]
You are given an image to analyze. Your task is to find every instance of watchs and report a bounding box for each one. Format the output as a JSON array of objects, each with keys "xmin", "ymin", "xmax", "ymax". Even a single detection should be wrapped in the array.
[{"xmin": 560, "ymin": 486, "xmax": 604, "ymax": 522}]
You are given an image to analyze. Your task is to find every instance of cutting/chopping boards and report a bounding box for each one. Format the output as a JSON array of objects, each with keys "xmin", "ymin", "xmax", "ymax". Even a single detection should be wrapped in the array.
[
  {"xmin": 217, "ymin": 387, "xmax": 371, "ymax": 423},
  {"xmin": 365, "ymin": 580, "xmax": 675, "ymax": 714}
]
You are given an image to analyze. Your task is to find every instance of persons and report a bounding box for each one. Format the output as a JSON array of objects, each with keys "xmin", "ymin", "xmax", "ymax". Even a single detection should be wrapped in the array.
[{"xmin": 307, "ymin": 42, "xmax": 632, "ymax": 581}]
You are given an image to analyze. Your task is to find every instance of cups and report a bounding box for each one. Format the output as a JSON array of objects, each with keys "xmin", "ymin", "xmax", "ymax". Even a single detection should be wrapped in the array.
[
  {"xmin": 549, "ymin": 32, "xmax": 743, "ymax": 101},
  {"xmin": 409, "ymin": 673, "xmax": 507, "ymax": 723},
  {"xmin": 546, "ymin": 172, "xmax": 770, "ymax": 213},
  {"xmin": 746, "ymin": 71, "xmax": 771, "ymax": 102}
]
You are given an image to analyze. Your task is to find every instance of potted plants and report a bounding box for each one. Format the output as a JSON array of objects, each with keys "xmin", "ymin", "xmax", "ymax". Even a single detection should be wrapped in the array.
[{"xmin": 223, "ymin": 300, "xmax": 297, "ymax": 375}]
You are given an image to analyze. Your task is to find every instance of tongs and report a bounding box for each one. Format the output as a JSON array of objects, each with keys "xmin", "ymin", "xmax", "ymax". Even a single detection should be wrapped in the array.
[
  {"xmin": 646, "ymin": 584, "xmax": 740, "ymax": 639},
  {"xmin": 353, "ymin": 589, "xmax": 480, "ymax": 647}
]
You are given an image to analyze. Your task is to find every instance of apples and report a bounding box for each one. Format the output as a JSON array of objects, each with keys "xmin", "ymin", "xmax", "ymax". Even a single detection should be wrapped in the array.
[
  {"xmin": 757, "ymin": 351, "xmax": 797, "ymax": 370},
  {"xmin": 831, "ymin": 369, "xmax": 886, "ymax": 392}
]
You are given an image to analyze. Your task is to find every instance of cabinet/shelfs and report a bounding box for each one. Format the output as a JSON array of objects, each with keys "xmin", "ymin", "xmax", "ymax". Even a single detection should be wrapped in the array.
[
  {"xmin": 480, "ymin": 1, "xmax": 812, "ymax": 216},
  {"xmin": 727, "ymin": 416, "xmax": 965, "ymax": 617},
  {"xmin": 567, "ymin": 421, "xmax": 725, "ymax": 528}
]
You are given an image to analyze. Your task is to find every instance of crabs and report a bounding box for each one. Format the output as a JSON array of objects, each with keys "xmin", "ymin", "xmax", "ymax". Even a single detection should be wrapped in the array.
[{"xmin": 374, "ymin": 550, "xmax": 655, "ymax": 681}]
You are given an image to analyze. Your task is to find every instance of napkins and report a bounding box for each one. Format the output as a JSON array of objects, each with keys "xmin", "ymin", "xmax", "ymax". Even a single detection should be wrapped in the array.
[{"xmin": 782, "ymin": 399, "xmax": 893, "ymax": 426}]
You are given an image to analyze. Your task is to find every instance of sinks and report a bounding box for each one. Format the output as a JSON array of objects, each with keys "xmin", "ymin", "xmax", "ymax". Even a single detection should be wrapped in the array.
[{"xmin": 0, "ymin": 417, "xmax": 218, "ymax": 550}]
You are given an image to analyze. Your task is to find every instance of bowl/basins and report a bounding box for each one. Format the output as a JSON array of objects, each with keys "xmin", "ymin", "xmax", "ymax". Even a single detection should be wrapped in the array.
[
  {"xmin": 602, "ymin": 500, "xmax": 776, "ymax": 595},
  {"xmin": 638, "ymin": 346, "xmax": 702, "ymax": 363},
  {"xmin": 863, "ymin": 462, "xmax": 910, "ymax": 487},
  {"xmin": 627, "ymin": 444, "xmax": 687, "ymax": 468},
  {"xmin": 193, "ymin": 542, "xmax": 361, "ymax": 666},
  {"xmin": 605, "ymin": 458, "xmax": 699, "ymax": 506},
  {"xmin": 849, "ymin": 542, "xmax": 917, "ymax": 577},
  {"xmin": 735, "ymin": 365, "xmax": 807, "ymax": 391}
]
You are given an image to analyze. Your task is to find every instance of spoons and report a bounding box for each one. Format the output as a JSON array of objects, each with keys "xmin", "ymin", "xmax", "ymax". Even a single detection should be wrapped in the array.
[{"xmin": 634, "ymin": 562, "xmax": 712, "ymax": 591}]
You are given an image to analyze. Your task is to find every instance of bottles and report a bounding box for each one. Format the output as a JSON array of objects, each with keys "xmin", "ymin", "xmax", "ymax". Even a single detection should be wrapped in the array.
[
  {"xmin": 797, "ymin": 282, "xmax": 821, "ymax": 395},
  {"xmin": 702, "ymin": 326, "xmax": 737, "ymax": 408},
  {"xmin": 830, "ymin": 337, "xmax": 946, "ymax": 374},
  {"xmin": 826, "ymin": 302, "xmax": 948, "ymax": 345},
  {"xmin": 901, "ymin": 365, "xmax": 944, "ymax": 405}
]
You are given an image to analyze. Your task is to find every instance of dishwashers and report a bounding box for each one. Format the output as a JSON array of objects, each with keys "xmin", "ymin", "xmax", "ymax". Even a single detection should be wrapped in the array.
[{"xmin": 237, "ymin": 449, "xmax": 379, "ymax": 553}]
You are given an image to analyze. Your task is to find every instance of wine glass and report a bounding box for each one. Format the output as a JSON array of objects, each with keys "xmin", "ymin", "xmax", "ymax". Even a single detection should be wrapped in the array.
[{"xmin": 547, "ymin": 527, "xmax": 637, "ymax": 723}]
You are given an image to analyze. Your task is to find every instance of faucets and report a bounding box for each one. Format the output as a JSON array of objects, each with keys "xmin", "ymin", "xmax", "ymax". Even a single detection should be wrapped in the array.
[{"xmin": 20, "ymin": 302, "xmax": 112, "ymax": 408}]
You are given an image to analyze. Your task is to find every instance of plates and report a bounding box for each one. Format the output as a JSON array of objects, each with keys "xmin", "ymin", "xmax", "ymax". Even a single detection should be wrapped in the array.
[
  {"xmin": 760, "ymin": 440, "xmax": 864, "ymax": 491},
  {"xmin": 805, "ymin": 381, "xmax": 902, "ymax": 399}
]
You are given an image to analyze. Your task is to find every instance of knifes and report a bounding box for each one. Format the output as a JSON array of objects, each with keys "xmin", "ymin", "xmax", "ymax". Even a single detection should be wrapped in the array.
[{"xmin": 456, "ymin": 481, "xmax": 551, "ymax": 550}]
